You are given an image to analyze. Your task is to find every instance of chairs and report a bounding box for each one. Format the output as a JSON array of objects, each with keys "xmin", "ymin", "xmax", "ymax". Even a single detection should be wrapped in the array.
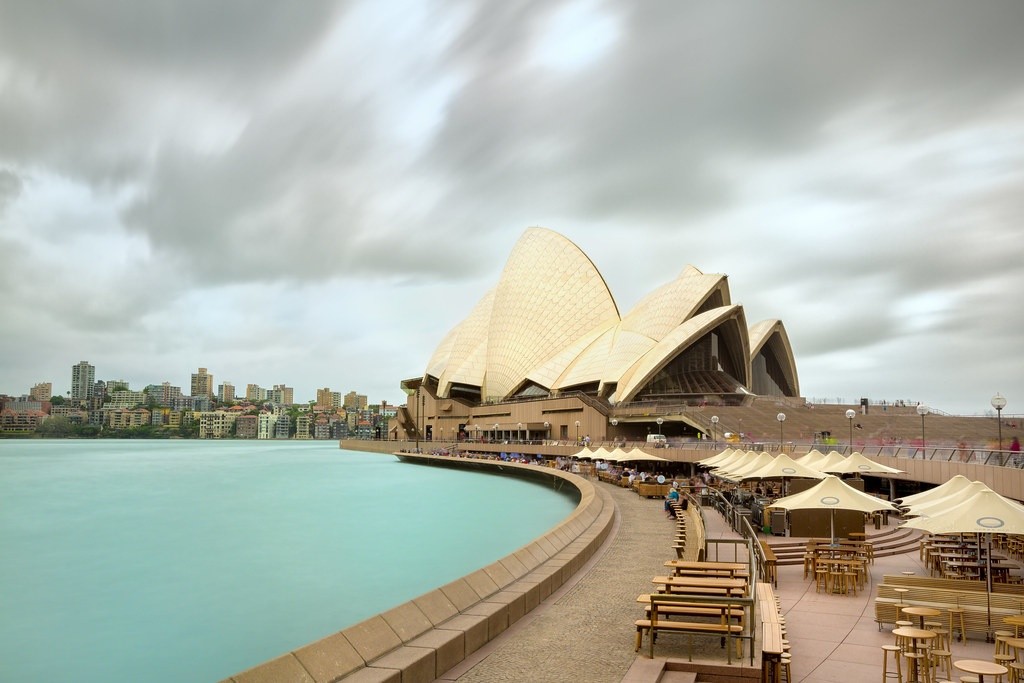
[
  {"xmin": 597, "ymin": 464, "xmax": 673, "ymax": 500},
  {"xmin": 664, "ymin": 490, "xmax": 707, "ymax": 562}
]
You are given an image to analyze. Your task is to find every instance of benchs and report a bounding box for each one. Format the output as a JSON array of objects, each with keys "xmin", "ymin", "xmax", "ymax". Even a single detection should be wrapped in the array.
[
  {"xmin": 874, "ymin": 597, "xmax": 1023, "ymax": 645},
  {"xmin": 882, "ymin": 574, "xmax": 1024, "ymax": 597},
  {"xmin": 634, "ymin": 620, "xmax": 744, "ymax": 659},
  {"xmin": 876, "ymin": 584, "xmax": 1024, "ymax": 612},
  {"xmin": 671, "ymin": 569, "xmax": 750, "ymax": 597},
  {"xmin": 643, "ymin": 605, "xmax": 745, "ymax": 642},
  {"xmin": 656, "ymin": 586, "xmax": 745, "ymax": 598}
]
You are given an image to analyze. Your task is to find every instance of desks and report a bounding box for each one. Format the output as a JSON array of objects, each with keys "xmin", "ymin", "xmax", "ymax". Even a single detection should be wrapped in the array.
[
  {"xmin": 664, "ymin": 560, "xmax": 746, "ymax": 579},
  {"xmin": 900, "ymin": 607, "xmax": 943, "ymax": 667},
  {"xmin": 756, "ymin": 582, "xmax": 775, "ymax": 602},
  {"xmin": 891, "ymin": 628, "xmax": 937, "ymax": 683},
  {"xmin": 803, "ymin": 531, "xmax": 874, "ymax": 594},
  {"xmin": 918, "ymin": 529, "xmax": 1024, "ymax": 585},
  {"xmin": 652, "ymin": 576, "xmax": 745, "ymax": 597},
  {"xmin": 758, "ymin": 540, "xmax": 778, "ymax": 591},
  {"xmin": 1003, "ymin": 615, "xmax": 1024, "ymax": 639},
  {"xmin": 636, "ymin": 594, "xmax": 745, "ymax": 646},
  {"xmin": 761, "ymin": 623, "xmax": 783, "ymax": 683},
  {"xmin": 759, "ymin": 600, "xmax": 779, "ymax": 624},
  {"xmin": 952, "ymin": 660, "xmax": 1009, "ymax": 683}
]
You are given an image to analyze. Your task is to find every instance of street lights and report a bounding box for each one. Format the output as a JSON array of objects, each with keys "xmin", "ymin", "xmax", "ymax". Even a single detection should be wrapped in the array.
[
  {"xmin": 991, "ymin": 392, "xmax": 1007, "ymax": 466},
  {"xmin": 518, "ymin": 423, "xmax": 523, "ymax": 445},
  {"xmin": 777, "ymin": 412, "xmax": 787, "ymax": 453},
  {"xmin": 495, "ymin": 424, "xmax": 499, "ymax": 444},
  {"xmin": 657, "ymin": 418, "xmax": 664, "ymax": 447},
  {"xmin": 845, "ymin": 409, "xmax": 856, "ymax": 455},
  {"xmin": 917, "ymin": 402, "xmax": 930, "ymax": 459},
  {"xmin": 711, "ymin": 415, "xmax": 720, "ymax": 450},
  {"xmin": 575, "ymin": 421, "xmax": 581, "ymax": 446},
  {"xmin": 544, "ymin": 422, "xmax": 550, "ymax": 446},
  {"xmin": 612, "ymin": 419, "xmax": 618, "ymax": 447},
  {"xmin": 355, "ymin": 424, "xmax": 480, "ymax": 443}
]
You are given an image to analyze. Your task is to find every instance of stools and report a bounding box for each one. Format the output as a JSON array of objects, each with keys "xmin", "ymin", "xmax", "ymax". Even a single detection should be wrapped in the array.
[
  {"xmin": 948, "ymin": 609, "xmax": 967, "ymax": 648},
  {"xmin": 829, "ymin": 571, "xmax": 843, "ymax": 597},
  {"xmin": 924, "ymin": 622, "xmax": 944, "ymax": 660},
  {"xmin": 881, "ymin": 645, "xmax": 903, "ymax": 683},
  {"xmin": 1008, "ymin": 641, "xmax": 1023, "ymax": 679},
  {"xmin": 1009, "ymin": 662, "xmax": 1024, "ymax": 683},
  {"xmin": 894, "ymin": 604, "xmax": 911, "ymax": 629},
  {"xmin": 993, "ymin": 655, "xmax": 1015, "ymax": 683},
  {"xmin": 894, "ymin": 620, "xmax": 914, "ymax": 659},
  {"xmin": 993, "ymin": 630, "xmax": 1015, "ymax": 656},
  {"xmin": 996, "ymin": 637, "xmax": 1014, "ymax": 668},
  {"xmin": 903, "ymin": 653, "xmax": 926, "ymax": 683},
  {"xmin": 902, "ymin": 571, "xmax": 916, "ymax": 576},
  {"xmin": 816, "ymin": 570, "xmax": 828, "ymax": 595},
  {"xmin": 775, "ymin": 594, "xmax": 791, "ymax": 654},
  {"xmin": 909, "ymin": 644, "xmax": 929, "ymax": 677},
  {"xmin": 895, "ymin": 588, "xmax": 910, "ymax": 602},
  {"xmin": 844, "ymin": 572, "xmax": 858, "ymax": 597},
  {"xmin": 779, "ymin": 653, "xmax": 792, "ymax": 681},
  {"xmin": 960, "ymin": 676, "xmax": 980, "ymax": 683},
  {"xmin": 930, "ymin": 629, "xmax": 953, "ymax": 672},
  {"xmin": 780, "ymin": 659, "xmax": 792, "ymax": 683},
  {"xmin": 930, "ymin": 650, "xmax": 952, "ymax": 683}
]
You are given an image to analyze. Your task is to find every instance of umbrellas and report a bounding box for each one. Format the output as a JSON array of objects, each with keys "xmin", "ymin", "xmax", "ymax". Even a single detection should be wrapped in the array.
[{"xmin": 689, "ymin": 447, "xmax": 1024, "ymax": 646}]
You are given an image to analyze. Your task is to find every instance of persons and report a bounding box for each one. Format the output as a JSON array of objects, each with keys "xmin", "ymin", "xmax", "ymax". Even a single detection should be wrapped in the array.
[
  {"xmin": 554, "ymin": 445, "xmax": 689, "ymax": 521},
  {"xmin": 577, "ymin": 434, "xmax": 666, "ymax": 449},
  {"xmin": 399, "ymin": 446, "xmax": 550, "ymax": 468},
  {"xmin": 960, "ymin": 426, "xmax": 1021, "ymax": 468},
  {"xmin": 712, "ymin": 429, "xmax": 929, "ymax": 456},
  {"xmin": 883, "ymin": 399, "xmax": 920, "ymax": 412}
]
